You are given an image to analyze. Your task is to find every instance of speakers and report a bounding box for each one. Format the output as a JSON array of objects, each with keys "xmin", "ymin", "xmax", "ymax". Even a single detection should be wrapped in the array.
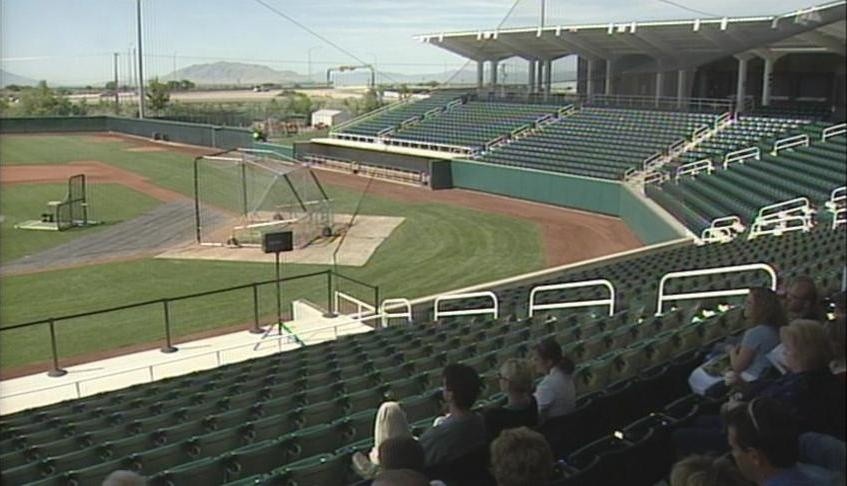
[{"xmin": 261, "ymin": 232, "xmax": 293, "ymax": 252}]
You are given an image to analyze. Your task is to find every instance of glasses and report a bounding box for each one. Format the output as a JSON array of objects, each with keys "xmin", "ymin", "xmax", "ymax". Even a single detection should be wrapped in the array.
[{"xmin": 747, "ymin": 393, "xmax": 785, "ymax": 434}]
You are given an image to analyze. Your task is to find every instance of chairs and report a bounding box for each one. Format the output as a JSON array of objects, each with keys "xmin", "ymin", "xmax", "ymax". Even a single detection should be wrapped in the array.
[
  {"xmin": 332, "ymin": 89, "xmax": 846, "ymax": 182},
  {"xmin": 645, "ymin": 183, "xmax": 846, "ymax": 241},
  {"xmin": 0, "ymin": 240, "xmax": 846, "ymax": 485}
]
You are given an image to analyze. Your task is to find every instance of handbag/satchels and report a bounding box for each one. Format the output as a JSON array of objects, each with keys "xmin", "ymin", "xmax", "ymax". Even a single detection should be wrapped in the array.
[{"xmin": 689, "ymin": 366, "xmax": 725, "ymax": 396}]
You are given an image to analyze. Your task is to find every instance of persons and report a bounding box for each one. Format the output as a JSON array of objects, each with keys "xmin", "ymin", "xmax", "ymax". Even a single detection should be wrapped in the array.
[
  {"xmin": 667, "ymin": 274, "xmax": 846, "ymax": 485},
  {"xmin": 353, "ymin": 336, "xmax": 580, "ymax": 486}
]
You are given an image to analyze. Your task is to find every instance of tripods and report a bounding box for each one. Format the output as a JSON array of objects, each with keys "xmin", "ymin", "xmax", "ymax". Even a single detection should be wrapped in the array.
[{"xmin": 254, "ymin": 253, "xmax": 306, "ymax": 350}]
